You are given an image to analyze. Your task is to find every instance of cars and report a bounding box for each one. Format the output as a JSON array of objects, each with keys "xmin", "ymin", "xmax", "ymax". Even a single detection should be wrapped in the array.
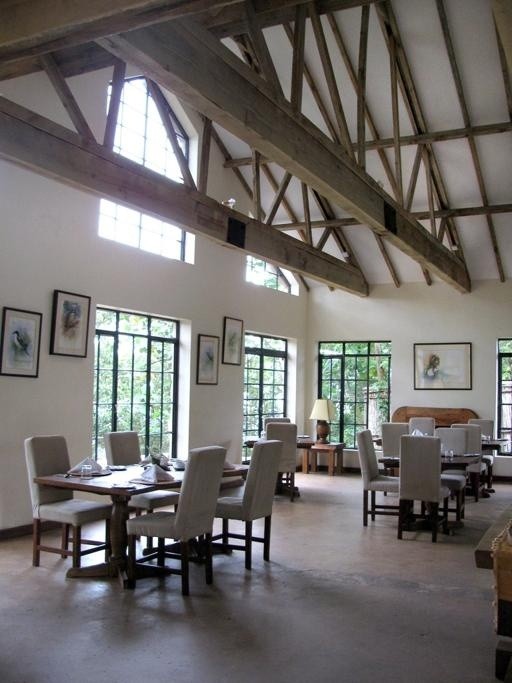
[
  {"xmin": 445, "ymin": 450, "xmax": 453, "ymax": 460},
  {"xmin": 80, "ymin": 465, "xmax": 92, "ymax": 479}
]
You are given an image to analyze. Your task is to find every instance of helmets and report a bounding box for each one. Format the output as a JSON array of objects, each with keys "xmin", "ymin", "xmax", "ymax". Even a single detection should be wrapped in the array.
[
  {"xmin": 129, "ymin": 479, "xmax": 178, "ymax": 486},
  {"xmin": 463, "ymin": 453, "xmax": 481, "ymax": 457},
  {"xmin": 66, "ymin": 470, "xmax": 113, "ymax": 476}
]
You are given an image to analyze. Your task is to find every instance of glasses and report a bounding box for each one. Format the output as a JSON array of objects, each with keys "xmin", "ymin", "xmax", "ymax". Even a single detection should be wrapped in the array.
[{"xmin": 308, "ymin": 399, "xmax": 336, "ymax": 444}]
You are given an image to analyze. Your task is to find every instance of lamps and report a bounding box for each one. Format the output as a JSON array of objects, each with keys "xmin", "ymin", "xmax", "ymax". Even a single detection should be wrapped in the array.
[
  {"xmin": 125, "ymin": 444, "xmax": 227, "ymax": 596},
  {"xmin": 103, "ymin": 431, "xmax": 180, "ymax": 548},
  {"xmin": 266, "ymin": 422, "xmax": 298, "ymax": 502},
  {"xmin": 468, "ymin": 419, "xmax": 494, "ymax": 489},
  {"xmin": 381, "ymin": 422, "xmax": 409, "ymax": 457},
  {"xmin": 263, "ymin": 417, "xmax": 291, "ymax": 436},
  {"xmin": 450, "ymin": 423, "xmax": 486, "ymax": 502},
  {"xmin": 215, "ymin": 439, "xmax": 284, "ymax": 570},
  {"xmin": 409, "ymin": 417, "xmax": 435, "ymax": 435},
  {"xmin": 357, "ymin": 429, "xmax": 399, "ymax": 526},
  {"xmin": 397, "ymin": 434, "xmax": 451, "ymax": 543},
  {"xmin": 435, "ymin": 428, "xmax": 466, "ymax": 521},
  {"xmin": 25, "ymin": 435, "xmax": 112, "ymax": 567}
]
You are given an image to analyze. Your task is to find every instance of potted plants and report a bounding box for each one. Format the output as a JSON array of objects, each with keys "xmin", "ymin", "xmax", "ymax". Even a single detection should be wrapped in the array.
[
  {"xmin": 49, "ymin": 289, "xmax": 91, "ymax": 358},
  {"xmin": 413, "ymin": 342, "xmax": 473, "ymax": 391},
  {"xmin": 196, "ymin": 334, "xmax": 220, "ymax": 385},
  {"xmin": 0, "ymin": 306, "xmax": 43, "ymax": 378},
  {"xmin": 222, "ymin": 316, "xmax": 244, "ymax": 366}
]
